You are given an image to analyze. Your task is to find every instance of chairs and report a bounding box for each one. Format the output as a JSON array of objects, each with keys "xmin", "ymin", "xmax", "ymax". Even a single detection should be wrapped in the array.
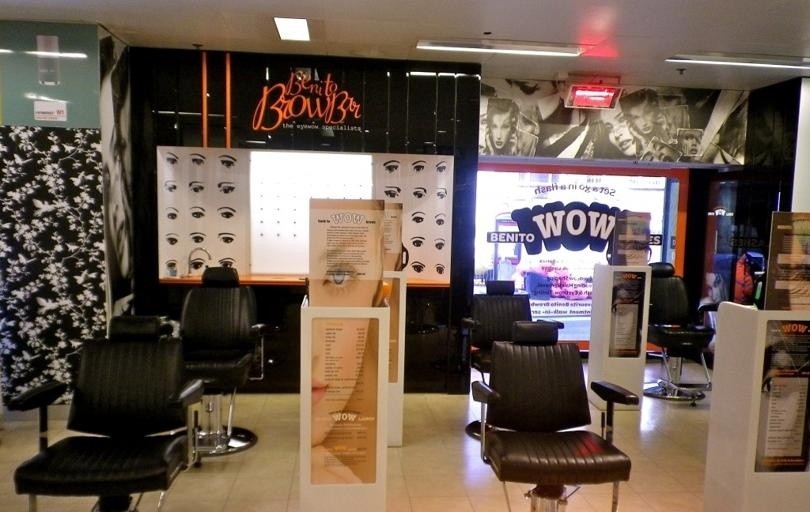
[
  {"xmin": 472, "ymin": 321, "xmax": 639, "ymax": 512},
  {"xmin": 460, "ymin": 281, "xmax": 564, "ymax": 441},
  {"xmin": 647, "ymin": 262, "xmax": 720, "ymax": 407},
  {"xmin": 6, "ymin": 315, "xmax": 205, "ymax": 512},
  {"xmin": 177, "ymin": 267, "xmax": 267, "ymax": 458}
]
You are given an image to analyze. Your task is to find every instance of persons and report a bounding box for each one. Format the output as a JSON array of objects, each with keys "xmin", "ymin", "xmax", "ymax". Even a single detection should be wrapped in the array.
[
  {"xmin": 110, "ymin": 50, "xmax": 133, "ymax": 204},
  {"xmin": 701, "ymin": 258, "xmax": 733, "ymax": 354},
  {"xmin": 486, "ymin": 94, "xmax": 523, "ymax": 157},
  {"xmin": 754, "ymin": 319, "xmax": 810, "ymax": 472},
  {"xmin": 99, "ymin": 50, "xmax": 133, "ymax": 287},
  {"xmin": 479, "ymin": 83, "xmax": 496, "ymax": 154},
  {"xmin": 377, "ymin": 202, "xmax": 404, "ymax": 308},
  {"xmin": 309, "ymin": 197, "xmax": 388, "ymax": 488},
  {"xmin": 609, "ymin": 271, "xmax": 642, "ymax": 357},
  {"xmin": 598, "ymin": 86, "xmax": 708, "ymax": 166}
]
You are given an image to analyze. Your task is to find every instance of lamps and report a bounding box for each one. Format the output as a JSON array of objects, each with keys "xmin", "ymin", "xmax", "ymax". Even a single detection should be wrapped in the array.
[
  {"xmin": 416, "ymin": 38, "xmax": 596, "ymax": 57},
  {"xmin": 664, "ymin": 51, "xmax": 810, "ymax": 70},
  {"xmin": 561, "ymin": 71, "xmax": 624, "ymax": 110}
]
